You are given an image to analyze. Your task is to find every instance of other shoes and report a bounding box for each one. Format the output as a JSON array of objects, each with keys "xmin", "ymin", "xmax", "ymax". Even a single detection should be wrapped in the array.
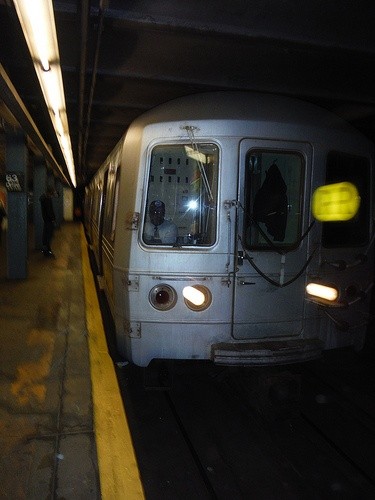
[{"xmin": 42, "ymin": 251, "xmax": 53, "ymax": 256}]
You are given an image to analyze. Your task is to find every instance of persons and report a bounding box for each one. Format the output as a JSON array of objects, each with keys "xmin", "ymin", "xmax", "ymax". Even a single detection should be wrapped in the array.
[
  {"xmin": 39, "ymin": 185, "xmax": 57, "ymax": 257},
  {"xmin": 142, "ymin": 200, "xmax": 178, "ymax": 244}
]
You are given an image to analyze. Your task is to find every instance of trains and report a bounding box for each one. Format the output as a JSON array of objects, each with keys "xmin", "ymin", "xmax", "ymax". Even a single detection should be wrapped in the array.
[{"xmin": 85, "ymin": 92, "xmax": 375, "ymax": 368}]
